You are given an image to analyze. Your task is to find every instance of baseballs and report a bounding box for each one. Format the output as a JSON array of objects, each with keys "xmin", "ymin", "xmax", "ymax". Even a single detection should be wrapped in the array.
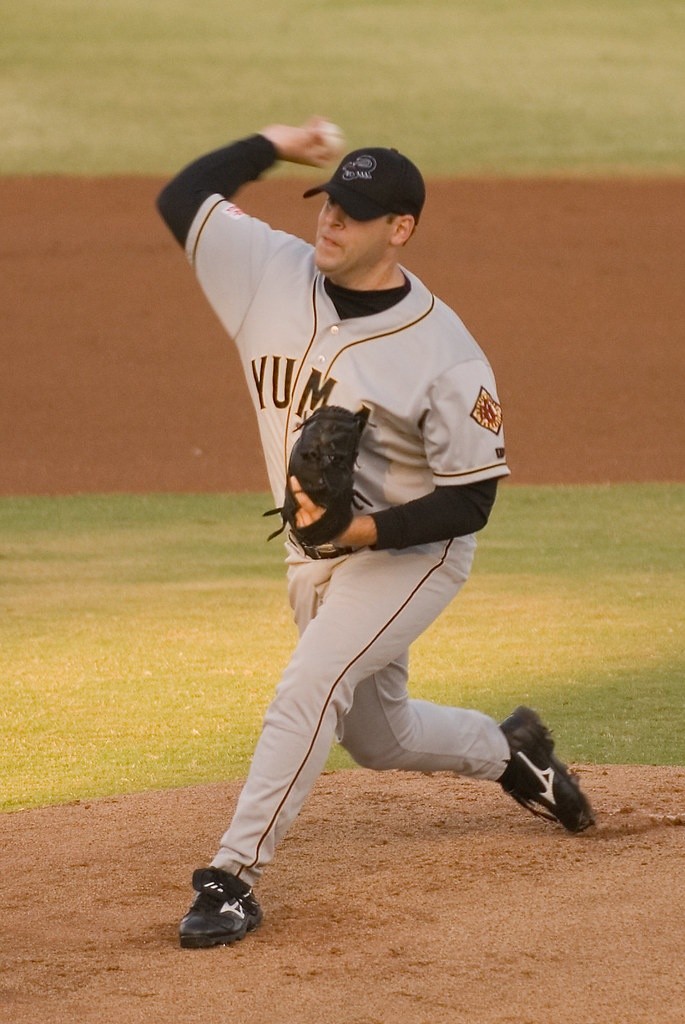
[{"xmin": 317, "ymin": 119, "xmax": 344, "ymax": 159}]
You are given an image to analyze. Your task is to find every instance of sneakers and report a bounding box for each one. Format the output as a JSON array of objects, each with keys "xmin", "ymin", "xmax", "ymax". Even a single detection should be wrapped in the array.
[
  {"xmin": 177, "ymin": 866, "xmax": 262, "ymax": 949},
  {"xmin": 497, "ymin": 705, "xmax": 595, "ymax": 833}
]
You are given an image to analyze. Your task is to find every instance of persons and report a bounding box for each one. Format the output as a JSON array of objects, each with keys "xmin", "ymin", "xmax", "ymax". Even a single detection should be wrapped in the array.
[{"xmin": 155, "ymin": 112, "xmax": 597, "ymax": 950}]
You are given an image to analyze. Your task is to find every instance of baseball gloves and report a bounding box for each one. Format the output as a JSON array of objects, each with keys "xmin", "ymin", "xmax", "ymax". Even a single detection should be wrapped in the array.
[{"xmin": 266, "ymin": 402, "xmax": 366, "ymax": 550}]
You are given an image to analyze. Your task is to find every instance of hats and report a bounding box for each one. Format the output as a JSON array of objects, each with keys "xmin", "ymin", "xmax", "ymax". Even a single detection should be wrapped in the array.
[{"xmin": 303, "ymin": 147, "xmax": 429, "ymax": 226}]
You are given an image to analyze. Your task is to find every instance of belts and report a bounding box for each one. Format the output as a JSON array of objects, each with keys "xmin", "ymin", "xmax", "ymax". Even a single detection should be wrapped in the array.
[{"xmin": 299, "ymin": 542, "xmax": 367, "ymax": 560}]
